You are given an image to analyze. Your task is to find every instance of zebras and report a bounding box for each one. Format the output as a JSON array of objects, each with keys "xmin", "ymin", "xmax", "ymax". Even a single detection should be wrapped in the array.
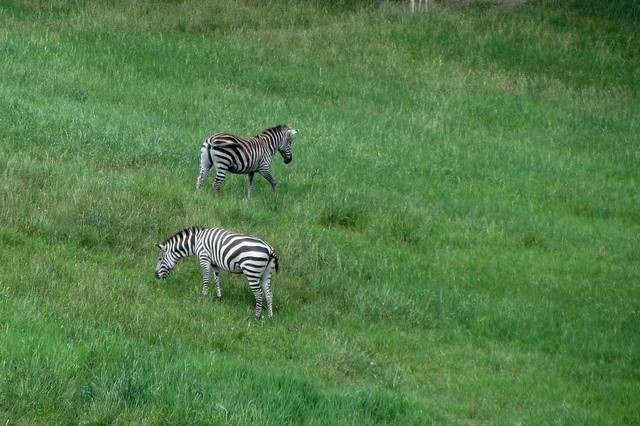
[
  {"xmin": 196, "ymin": 124, "xmax": 300, "ymax": 198},
  {"xmin": 155, "ymin": 224, "xmax": 279, "ymax": 320}
]
